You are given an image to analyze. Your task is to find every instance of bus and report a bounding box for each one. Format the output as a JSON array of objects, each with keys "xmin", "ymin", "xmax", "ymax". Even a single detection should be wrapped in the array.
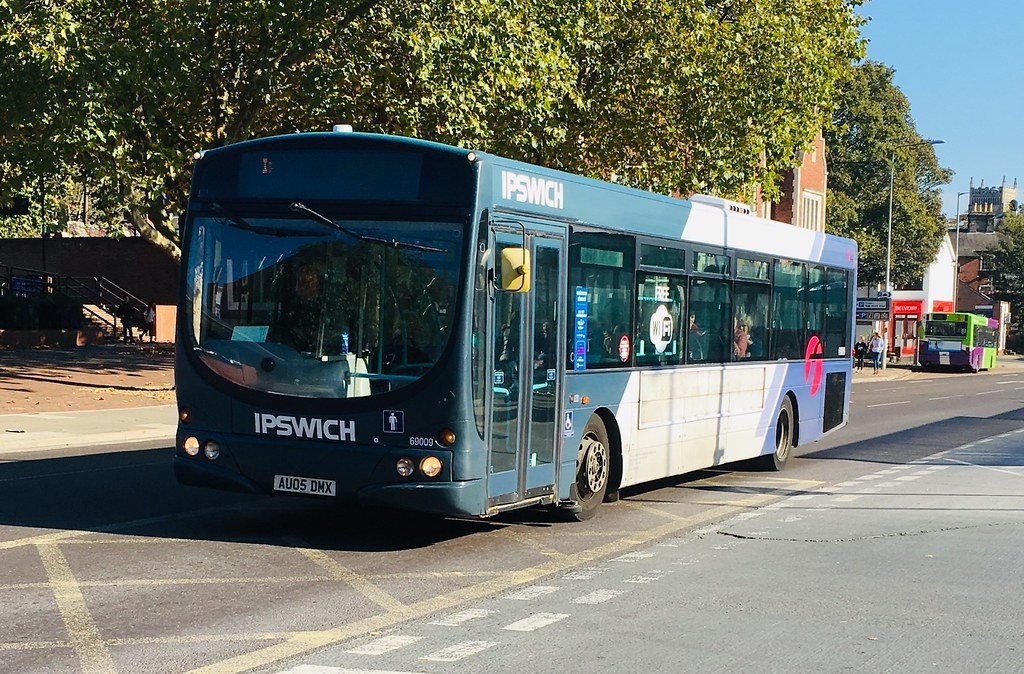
[
  {"xmin": 918, "ymin": 312, "xmax": 999, "ymax": 373},
  {"xmin": 174, "ymin": 124, "xmax": 858, "ymax": 532}
]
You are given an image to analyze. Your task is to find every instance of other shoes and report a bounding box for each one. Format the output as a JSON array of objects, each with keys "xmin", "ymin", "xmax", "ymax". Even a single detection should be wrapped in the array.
[
  {"xmin": 860, "ymin": 370, "xmax": 863, "ymax": 373},
  {"xmin": 873, "ymin": 371, "xmax": 879, "ymax": 375},
  {"xmin": 856, "ymin": 369, "xmax": 859, "ymax": 373}
]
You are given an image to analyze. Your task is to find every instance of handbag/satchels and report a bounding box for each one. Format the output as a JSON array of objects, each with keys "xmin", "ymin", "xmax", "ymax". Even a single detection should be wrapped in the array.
[{"xmin": 869, "ymin": 341, "xmax": 874, "ymax": 349}]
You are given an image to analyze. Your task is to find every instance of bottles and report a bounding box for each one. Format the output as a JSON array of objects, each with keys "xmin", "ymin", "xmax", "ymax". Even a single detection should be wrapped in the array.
[{"xmin": 339, "ymin": 331, "xmax": 349, "ymax": 355}]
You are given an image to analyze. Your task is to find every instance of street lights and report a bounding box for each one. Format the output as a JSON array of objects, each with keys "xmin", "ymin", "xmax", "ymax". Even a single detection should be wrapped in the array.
[
  {"xmin": 952, "ymin": 191, "xmax": 970, "ymax": 312},
  {"xmin": 884, "ymin": 139, "xmax": 946, "ymax": 291}
]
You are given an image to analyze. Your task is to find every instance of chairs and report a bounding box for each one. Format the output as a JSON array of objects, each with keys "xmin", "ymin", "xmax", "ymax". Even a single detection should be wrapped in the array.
[
  {"xmin": 778, "ymin": 306, "xmax": 803, "ymax": 359},
  {"xmin": 322, "ymin": 281, "xmax": 356, "ymax": 330}
]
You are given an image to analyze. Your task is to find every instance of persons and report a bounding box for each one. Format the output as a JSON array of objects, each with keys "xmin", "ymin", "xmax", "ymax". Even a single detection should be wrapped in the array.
[
  {"xmin": 866, "ymin": 333, "xmax": 885, "ymax": 375},
  {"xmin": 116, "ymin": 294, "xmax": 136, "ymax": 345},
  {"xmin": 139, "ymin": 300, "xmax": 156, "ymax": 344},
  {"xmin": 272, "ymin": 261, "xmax": 322, "ymax": 354},
  {"xmin": 498, "ymin": 309, "xmax": 557, "ymax": 403},
  {"xmin": 601, "ymin": 296, "xmax": 753, "ymax": 366},
  {"xmin": 854, "ymin": 335, "xmax": 868, "ymax": 373}
]
